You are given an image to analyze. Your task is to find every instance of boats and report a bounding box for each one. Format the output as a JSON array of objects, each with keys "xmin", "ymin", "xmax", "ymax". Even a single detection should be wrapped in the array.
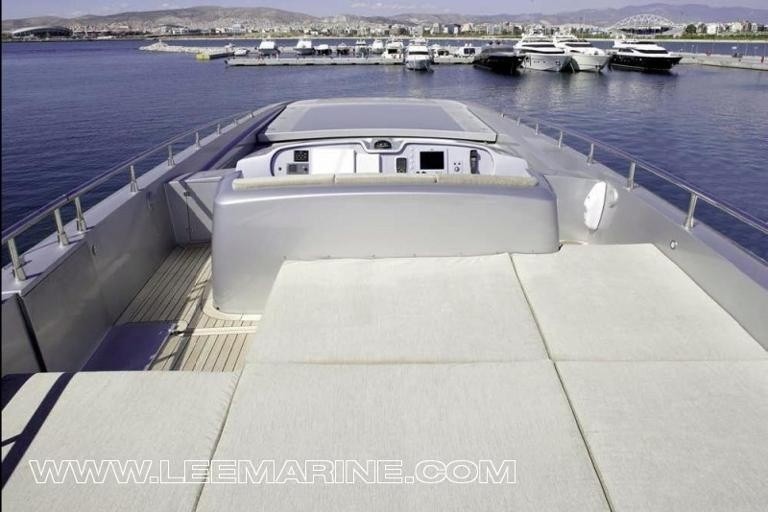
[
  {"xmin": 196, "ymin": 38, "xmax": 482, "ymax": 72},
  {"xmin": 513, "ymin": 26, "xmax": 572, "ymax": 73},
  {"xmin": 474, "ymin": 40, "xmax": 525, "ymax": 75},
  {"xmin": 553, "ymin": 34, "xmax": 613, "ymax": 74},
  {"xmin": 607, "ymin": 39, "xmax": 682, "ymax": 74},
  {"xmin": 2, "ymin": 95, "xmax": 768, "ymax": 512}
]
